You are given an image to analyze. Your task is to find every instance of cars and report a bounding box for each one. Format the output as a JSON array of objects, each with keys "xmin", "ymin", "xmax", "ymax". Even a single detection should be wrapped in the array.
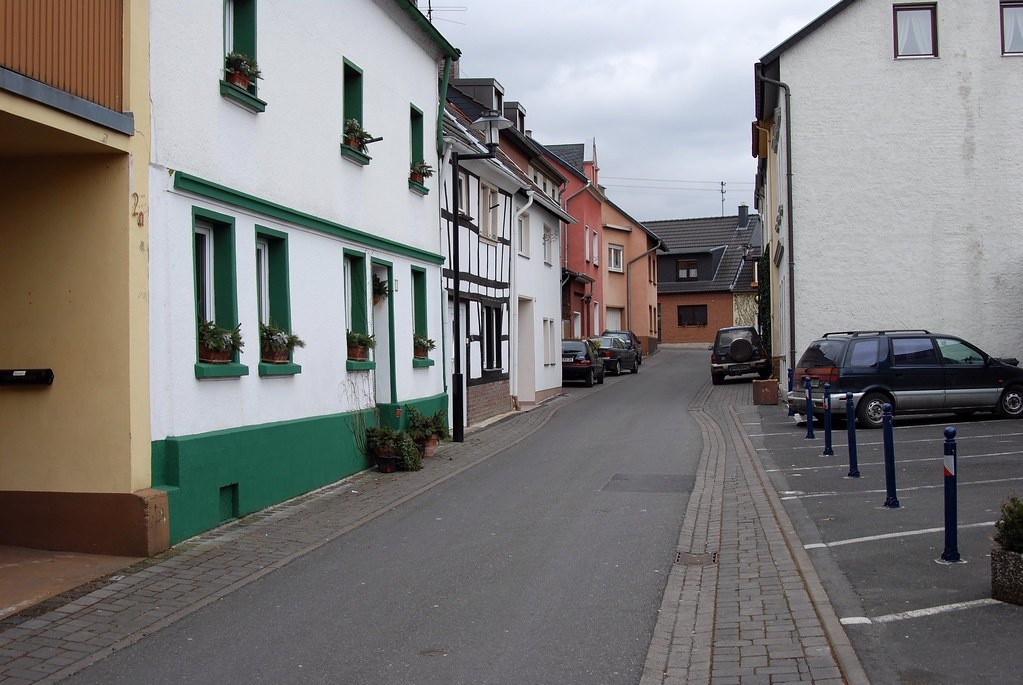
[
  {"xmin": 590, "ymin": 337, "xmax": 638, "ymax": 376},
  {"xmin": 562, "ymin": 339, "xmax": 604, "ymax": 387}
]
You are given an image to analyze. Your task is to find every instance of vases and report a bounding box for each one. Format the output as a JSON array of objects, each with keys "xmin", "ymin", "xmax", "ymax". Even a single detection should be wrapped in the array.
[
  {"xmin": 414, "ymin": 347, "xmax": 430, "ymax": 359},
  {"xmin": 415, "ymin": 434, "xmax": 440, "ymax": 457},
  {"xmin": 264, "ymin": 346, "xmax": 290, "ymax": 362},
  {"xmin": 350, "ymin": 346, "xmax": 372, "ymax": 360},
  {"xmin": 201, "ymin": 348, "xmax": 233, "ymax": 362},
  {"xmin": 345, "ymin": 136, "xmax": 363, "ymax": 151},
  {"xmin": 411, "ymin": 174, "xmax": 425, "ymax": 185},
  {"xmin": 379, "ymin": 454, "xmax": 405, "ymax": 474},
  {"xmin": 231, "ymin": 72, "xmax": 252, "ymax": 90}
]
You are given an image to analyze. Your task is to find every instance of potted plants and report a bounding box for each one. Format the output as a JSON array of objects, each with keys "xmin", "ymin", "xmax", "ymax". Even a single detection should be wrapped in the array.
[{"xmin": 372, "ymin": 273, "xmax": 391, "ymax": 306}]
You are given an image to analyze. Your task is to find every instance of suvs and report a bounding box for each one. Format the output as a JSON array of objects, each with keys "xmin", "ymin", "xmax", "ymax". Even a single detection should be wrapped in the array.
[
  {"xmin": 788, "ymin": 329, "xmax": 1023, "ymax": 428},
  {"xmin": 709, "ymin": 326, "xmax": 772, "ymax": 385},
  {"xmin": 602, "ymin": 329, "xmax": 642, "ymax": 365}
]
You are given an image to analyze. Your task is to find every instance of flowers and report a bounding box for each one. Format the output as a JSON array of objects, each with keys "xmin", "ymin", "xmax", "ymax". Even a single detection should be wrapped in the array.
[
  {"xmin": 199, "ymin": 322, "xmax": 245, "ymax": 354},
  {"xmin": 260, "ymin": 322, "xmax": 305, "ymax": 350},
  {"xmin": 409, "ymin": 161, "xmax": 436, "ymax": 177},
  {"xmin": 366, "ymin": 427, "xmax": 424, "ymax": 471},
  {"xmin": 222, "ymin": 51, "xmax": 263, "ymax": 81},
  {"xmin": 414, "ymin": 335, "xmax": 439, "ymax": 352},
  {"xmin": 344, "ymin": 118, "xmax": 375, "ymax": 152},
  {"xmin": 402, "ymin": 405, "xmax": 452, "ymax": 442}
]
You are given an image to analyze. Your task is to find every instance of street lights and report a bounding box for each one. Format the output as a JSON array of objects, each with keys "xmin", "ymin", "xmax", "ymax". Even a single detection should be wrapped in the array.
[{"xmin": 452, "ymin": 109, "xmax": 513, "ymax": 444}]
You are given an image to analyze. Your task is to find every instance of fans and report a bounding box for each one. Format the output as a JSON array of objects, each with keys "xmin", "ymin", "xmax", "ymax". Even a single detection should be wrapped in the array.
[{"xmin": 347, "ymin": 328, "xmax": 379, "ymax": 350}]
